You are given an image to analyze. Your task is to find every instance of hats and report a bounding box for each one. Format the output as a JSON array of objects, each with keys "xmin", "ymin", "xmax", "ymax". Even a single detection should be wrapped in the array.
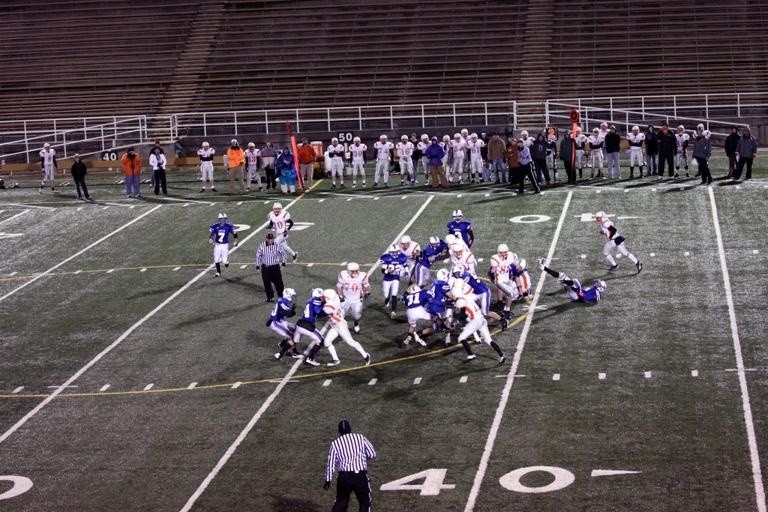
[{"xmin": 266, "ymin": 233, "xmax": 273, "ymax": 239}]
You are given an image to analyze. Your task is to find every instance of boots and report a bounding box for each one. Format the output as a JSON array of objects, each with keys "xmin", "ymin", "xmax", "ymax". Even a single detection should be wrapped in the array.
[
  {"xmin": 630, "ymin": 166, "xmax": 634, "ymax": 178},
  {"xmin": 639, "ymin": 165, "xmax": 643, "ymax": 177}
]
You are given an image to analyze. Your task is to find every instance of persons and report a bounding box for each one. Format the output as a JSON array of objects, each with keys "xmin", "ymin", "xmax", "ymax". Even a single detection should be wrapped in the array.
[
  {"xmin": 372, "ymin": 122, "xmax": 712, "ymax": 197},
  {"xmin": 274, "ymin": 288, "xmax": 324, "ymax": 367},
  {"xmin": 335, "ymin": 261, "xmax": 372, "ymax": 334},
  {"xmin": 197, "ymin": 136, "xmax": 367, "ymax": 196},
  {"xmin": 122, "ymin": 140, "xmax": 168, "ymax": 198},
  {"xmin": 265, "ymin": 201, "xmax": 298, "ymax": 267},
  {"xmin": 322, "ymin": 420, "xmax": 378, "ymax": 511},
  {"xmin": 267, "ymin": 285, "xmax": 300, "ymax": 360},
  {"xmin": 539, "ymin": 257, "xmax": 608, "ymax": 304},
  {"xmin": 378, "ymin": 209, "xmax": 534, "ymax": 364},
  {"xmin": 255, "ymin": 232, "xmax": 288, "ymax": 303},
  {"xmin": 596, "ymin": 210, "xmax": 643, "ymax": 274},
  {"xmin": 40, "ymin": 142, "xmax": 58, "ymax": 192},
  {"xmin": 322, "ymin": 288, "xmax": 371, "ymax": 367},
  {"xmin": 71, "ymin": 153, "xmax": 89, "ymax": 198},
  {"xmin": 724, "ymin": 126, "xmax": 757, "ymax": 181},
  {"xmin": 207, "ymin": 210, "xmax": 239, "ymax": 278}
]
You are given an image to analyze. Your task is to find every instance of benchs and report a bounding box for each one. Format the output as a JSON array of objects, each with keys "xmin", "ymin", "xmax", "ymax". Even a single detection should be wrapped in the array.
[{"xmin": 0, "ymin": 0, "xmax": 767, "ymax": 159}]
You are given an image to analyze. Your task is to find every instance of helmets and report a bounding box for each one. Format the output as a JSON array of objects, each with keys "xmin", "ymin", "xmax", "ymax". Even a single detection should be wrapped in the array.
[
  {"xmin": 380, "ymin": 134, "xmax": 408, "ymax": 144},
  {"xmin": 632, "ymin": 126, "xmax": 640, "ymax": 134},
  {"xmin": 497, "ymin": 244, "xmax": 508, "ymax": 259},
  {"xmin": 248, "ymin": 142, "xmax": 256, "ymax": 151},
  {"xmin": 390, "ymin": 236, "xmax": 411, "ymax": 256},
  {"xmin": 339, "ymin": 420, "xmax": 350, "ymax": 434},
  {"xmin": 521, "ymin": 130, "xmax": 528, "ymax": 138},
  {"xmin": 202, "ymin": 142, "xmax": 210, "ymax": 150},
  {"xmin": 678, "ymin": 125, "xmax": 685, "ymax": 134},
  {"xmin": 332, "ymin": 137, "xmax": 361, "ymax": 146},
  {"xmin": 283, "ymin": 288, "xmax": 296, "ymax": 298},
  {"xmin": 593, "ymin": 122, "xmax": 608, "ymax": 136},
  {"xmin": 312, "ymin": 263, "xmax": 359, "ymax": 302},
  {"xmin": 594, "ymin": 280, "xmax": 606, "ymax": 292},
  {"xmin": 273, "ymin": 202, "xmax": 282, "ymax": 214},
  {"xmin": 429, "ymin": 210, "xmax": 465, "ymax": 258},
  {"xmin": 437, "ymin": 265, "xmax": 465, "ymax": 298},
  {"xmin": 218, "ymin": 213, "xmax": 227, "ymax": 219},
  {"xmin": 595, "ymin": 211, "xmax": 606, "ymax": 224},
  {"xmin": 420, "ymin": 128, "xmax": 477, "ymax": 145}
]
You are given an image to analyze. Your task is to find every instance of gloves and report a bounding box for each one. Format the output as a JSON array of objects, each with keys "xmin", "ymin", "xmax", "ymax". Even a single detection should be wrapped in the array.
[{"xmin": 283, "ymin": 229, "xmax": 288, "ymax": 236}]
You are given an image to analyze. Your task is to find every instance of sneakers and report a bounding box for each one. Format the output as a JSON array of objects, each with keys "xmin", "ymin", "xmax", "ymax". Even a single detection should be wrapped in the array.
[
  {"xmin": 539, "ymin": 258, "xmax": 544, "ymax": 269},
  {"xmin": 495, "ymin": 301, "xmax": 513, "ymax": 331},
  {"xmin": 498, "ymin": 354, "xmax": 506, "ymax": 363},
  {"xmin": 353, "ymin": 321, "xmax": 359, "ymax": 333},
  {"xmin": 609, "ymin": 264, "xmax": 618, "ymax": 271},
  {"xmin": 401, "ymin": 179, "xmax": 429, "ymax": 186},
  {"xmin": 463, "ymin": 355, "xmax": 476, "ymax": 364},
  {"xmin": 404, "ymin": 331, "xmax": 452, "ymax": 347},
  {"xmin": 331, "ymin": 183, "xmax": 377, "ymax": 190},
  {"xmin": 385, "ymin": 298, "xmax": 397, "ymax": 318},
  {"xmin": 449, "ymin": 178, "xmax": 483, "ymax": 184},
  {"xmin": 275, "ymin": 339, "xmax": 340, "ymax": 367},
  {"xmin": 365, "ymin": 353, "xmax": 370, "ymax": 365},
  {"xmin": 293, "ymin": 251, "xmax": 298, "ymax": 263},
  {"xmin": 636, "ymin": 260, "xmax": 642, "ymax": 273}
]
